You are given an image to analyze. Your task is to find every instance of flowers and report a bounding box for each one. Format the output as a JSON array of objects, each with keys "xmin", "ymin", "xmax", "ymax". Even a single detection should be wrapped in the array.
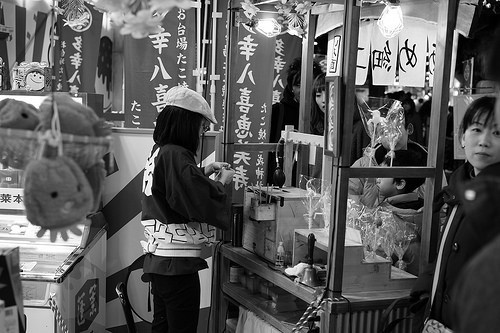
[{"xmin": 49, "ymin": 0, "xmax": 315, "ymax": 40}]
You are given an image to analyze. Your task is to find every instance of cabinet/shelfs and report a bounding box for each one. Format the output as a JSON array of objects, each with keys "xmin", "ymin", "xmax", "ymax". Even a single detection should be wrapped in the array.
[{"xmin": 208, "ymin": 241, "xmax": 421, "ymax": 333}]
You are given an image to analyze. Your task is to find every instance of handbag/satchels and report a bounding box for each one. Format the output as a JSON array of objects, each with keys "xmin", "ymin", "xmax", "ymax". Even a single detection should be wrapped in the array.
[{"xmin": 421, "ymin": 319, "xmax": 454, "ymax": 333}]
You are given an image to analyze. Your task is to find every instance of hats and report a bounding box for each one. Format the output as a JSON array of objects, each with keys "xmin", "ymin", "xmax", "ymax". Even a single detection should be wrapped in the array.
[{"xmin": 164, "ymin": 86, "xmax": 218, "ymax": 124}]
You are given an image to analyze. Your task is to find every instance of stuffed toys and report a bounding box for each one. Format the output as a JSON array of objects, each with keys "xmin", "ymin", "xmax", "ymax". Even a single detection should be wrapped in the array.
[
  {"xmin": 25, "ymin": 156, "xmax": 93, "ymax": 242},
  {"xmin": 23, "ymin": 67, "xmax": 49, "ymax": 92},
  {"xmin": 0, "ymin": 95, "xmax": 38, "ymax": 131},
  {"xmin": 40, "ymin": 95, "xmax": 111, "ymax": 139}
]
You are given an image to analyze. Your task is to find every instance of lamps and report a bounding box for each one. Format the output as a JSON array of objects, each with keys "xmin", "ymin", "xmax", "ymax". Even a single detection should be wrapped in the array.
[
  {"xmin": 377, "ymin": 0, "xmax": 405, "ymax": 39},
  {"xmin": 67, "ymin": 0, "xmax": 92, "ymax": 33},
  {"xmin": 244, "ymin": 17, "xmax": 281, "ymax": 39}
]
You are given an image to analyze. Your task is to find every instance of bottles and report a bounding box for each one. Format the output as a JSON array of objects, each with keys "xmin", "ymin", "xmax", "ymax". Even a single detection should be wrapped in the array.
[
  {"xmin": 275, "ymin": 242, "xmax": 292, "ymax": 270},
  {"xmin": 229, "ymin": 265, "xmax": 275, "ymax": 300},
  {"xmin": 223, "ymin": 204, "xmax": 243, "ymax": 247}
]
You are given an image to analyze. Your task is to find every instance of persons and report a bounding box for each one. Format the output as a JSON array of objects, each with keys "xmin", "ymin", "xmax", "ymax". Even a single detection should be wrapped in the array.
[
  {"xmin": 138, "ymin": 85, "xmax": 236, "ymax": 333},
  {"xmin": 267, "ymin": 56, "xmax": 323, "ymax": 187},
  {"xmin": 309, "ymin": 72, "xmax": 326, "ymax": 135},
  {"xmin": 347, "ymin": 105, "xmax": 447, "ymax": 237},
  {"xmin": 377, "ymin": 150, "xmax": 422, "ymax": 241},
  {"xmin": 403, "ymin": 94, "xmax": 500, "ymax": 333}
]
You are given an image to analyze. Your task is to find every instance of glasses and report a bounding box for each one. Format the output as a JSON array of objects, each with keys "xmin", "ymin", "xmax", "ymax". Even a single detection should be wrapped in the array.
[{"xmin": 200, "ymin": 121, "xmax": 210, "ymax": 132}]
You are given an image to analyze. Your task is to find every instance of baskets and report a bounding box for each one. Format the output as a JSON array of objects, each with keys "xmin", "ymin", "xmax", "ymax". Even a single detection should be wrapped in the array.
[{"xmin": 0, "ymin": 127, "xmax": 109, "ymax": 173}]
